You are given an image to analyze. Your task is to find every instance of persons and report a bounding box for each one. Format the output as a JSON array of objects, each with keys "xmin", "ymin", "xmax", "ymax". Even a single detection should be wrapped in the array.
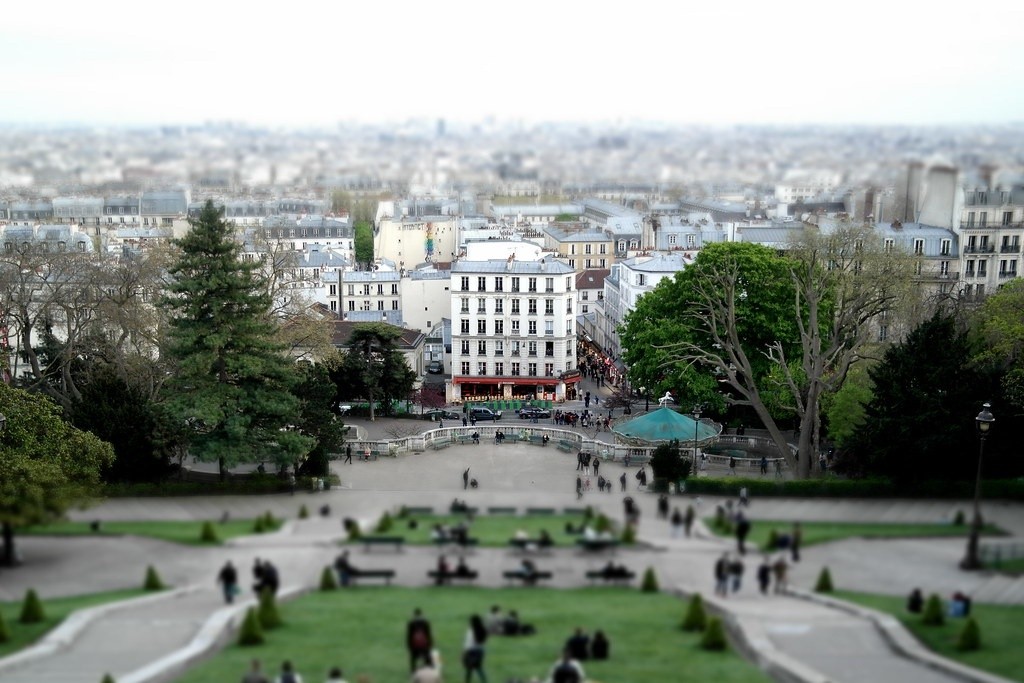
[
  {"xmin": 576, "ymin": 339, "xmax": 624, "ymax": 388},
  {"xmin": 219, "ymin": 388, "xmax": 982, "ymax": 683}
]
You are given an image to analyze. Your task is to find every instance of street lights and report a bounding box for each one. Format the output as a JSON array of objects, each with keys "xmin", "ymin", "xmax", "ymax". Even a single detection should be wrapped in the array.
[
  {"xmin": 962, "ymin": 400, "xmax": 998, "ymax": 571},
  {"xmin": 690, "ymin": 403, "xmax": 704, "ymax": 477}
]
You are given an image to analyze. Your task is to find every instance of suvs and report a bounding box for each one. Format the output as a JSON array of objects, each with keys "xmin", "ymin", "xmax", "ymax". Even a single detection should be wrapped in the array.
[
  {"xmin": 429, "ymin": 360, "xmax": 442, "ymax": 374},
  {"xmin": 470, "ymin": 407, "xmax": 502, "ymax": 420}
]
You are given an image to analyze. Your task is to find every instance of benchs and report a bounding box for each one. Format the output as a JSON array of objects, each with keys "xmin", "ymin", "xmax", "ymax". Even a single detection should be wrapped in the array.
[
  {"xmin": 458, "ymin": 435, "xmax": 479, "ymax": 444},
  {"xmin": 356, "ymin": 451, "xmax": 380, "ymax": 461},
  {"xmin": 528, "ymin": 435, "xmax": 550, "ymax": 446},
  {"xmin": 495, "ymin": 434, "xmax": 518, "ymax": 445},
  {"xmin": 348, "ymin": 570, "xmax": 395, "ymax": 585},
  {"xmin": 625, "ymin": 456, "xmax": 650, "ymax": 467},
  {"xmin": 359, "ymin": 537, "xmax": 403, "ymax": 553},
  {"xmin": 503, "ymin": 572, "xmax": 552, "ymax": 585},
  {"xmin": 428, "ymin": 571, "xmax": 478, "ymax": 586},
  {"xmin": 434, "ymin": 537, "xmax": 480, "ymax": 555},
  {"xmin": 586, "ymin": 571, "xmax": 634, "ymax": 585},
  {"xmin": 572, "ymin": 539, "xmax": 620, "ymax": 554},
  {"xmin": 433, "ymin": 438, "xmax": 452, "ymax": 450},
  {"xmin": 508, "ymin": 538, "xmax": 555, "ymax": 556},
  {"xmin": 556, "ymin": 440, "xmax": 573, "ymax": 453}
]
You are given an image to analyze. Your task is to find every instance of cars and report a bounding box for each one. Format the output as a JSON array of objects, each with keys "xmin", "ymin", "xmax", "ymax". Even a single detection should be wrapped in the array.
[
  {"xmin": 425, "ymin": 408, "xmax": 459, "ymax": 421},
  {"xmin": 519, "ymin": 406, "xmax": 551, "ymax": 419}
]
